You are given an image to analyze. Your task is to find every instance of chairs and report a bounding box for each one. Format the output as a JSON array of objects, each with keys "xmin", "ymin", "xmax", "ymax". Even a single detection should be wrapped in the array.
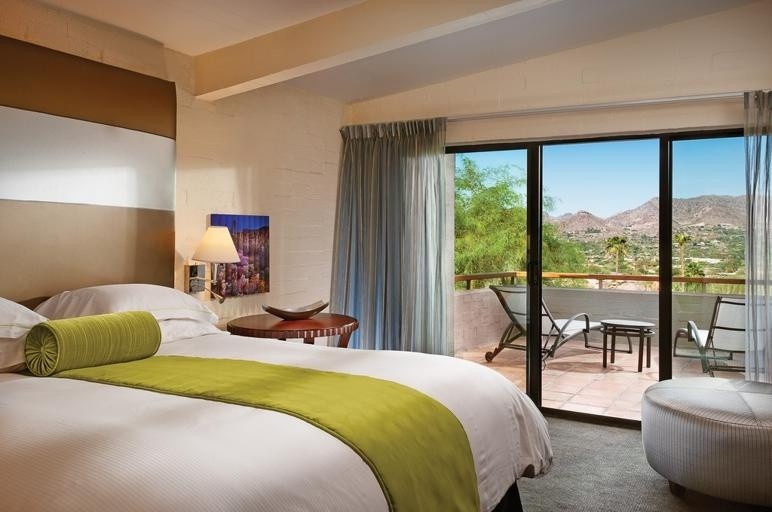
[
  {"xmin": 673, "ymin": 293, "xmax": 771, "ymax": 377},
  {"xmin": 484, "ymin": 284, "xmax": 632, "ymax": 370}
]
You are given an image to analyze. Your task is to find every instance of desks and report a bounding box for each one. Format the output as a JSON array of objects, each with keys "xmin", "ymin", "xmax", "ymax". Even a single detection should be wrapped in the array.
[
  {"xmin": 227, "ymin": 314, "xmax": 360, "ymax": 348},
  {"xmin": 599, "ymin": 320, "xmax": 655, "ymax": 372}
]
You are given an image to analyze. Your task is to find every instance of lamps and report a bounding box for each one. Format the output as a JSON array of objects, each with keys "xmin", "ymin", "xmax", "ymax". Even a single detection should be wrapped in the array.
[{"xmin": 183, "ymin": 225, "xmax": 242, "ymax": 304}]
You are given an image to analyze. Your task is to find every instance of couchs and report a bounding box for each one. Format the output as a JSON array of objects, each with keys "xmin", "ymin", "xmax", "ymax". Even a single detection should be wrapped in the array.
[{"xmin": 641, "ymin": 378, "xmax": 772, "ymax": 512}]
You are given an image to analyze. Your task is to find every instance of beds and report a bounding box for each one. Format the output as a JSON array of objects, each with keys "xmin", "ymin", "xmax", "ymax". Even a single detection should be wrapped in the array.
[{"xmin": 0, "ymin": 292, "xmax": 554, "ymax": 512}]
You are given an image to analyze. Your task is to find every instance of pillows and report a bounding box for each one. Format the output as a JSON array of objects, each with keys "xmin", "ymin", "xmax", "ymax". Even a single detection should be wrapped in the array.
[
  {"xmin": 0, "ymin": 338, "xmax": 28, "ymax": 372},
  {"xmin": 151, "ymin": 316, "xmax": 232, "ymax": 341},
  {"xmin": 0, "ymin": 296, "xmax": 50, "ymax": 340},
  {"xmin": 31, "ymin": 283, "xmax": 220, "ymax": 326}
]
